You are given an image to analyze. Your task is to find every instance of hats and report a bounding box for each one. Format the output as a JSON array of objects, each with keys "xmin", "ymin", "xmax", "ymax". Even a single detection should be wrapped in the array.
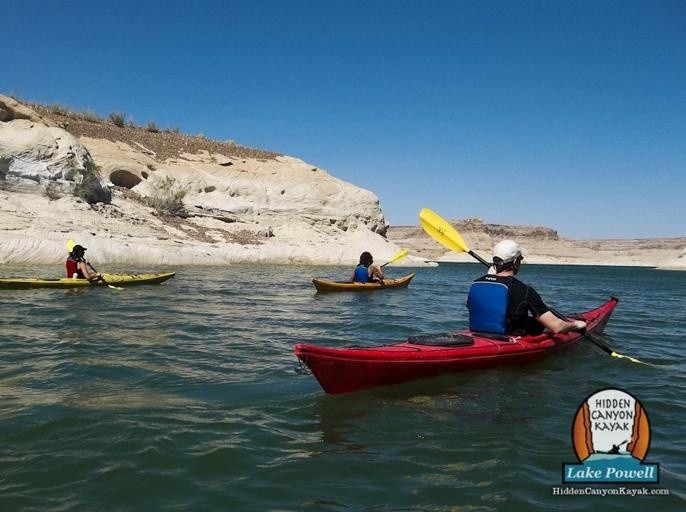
[
  {"xmin": 492, "ymin": 239, "xmax": 523, "ymax": 266},
  {"xmin": 73, "ymin": 245, "xmax": 87, "ymax": 252}
]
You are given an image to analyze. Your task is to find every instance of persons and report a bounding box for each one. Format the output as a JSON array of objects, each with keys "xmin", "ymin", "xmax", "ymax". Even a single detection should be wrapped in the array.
[
  {"xmin": 65, "ymin": 245, "xmax": 102, "ymax": 281},
  {"xmin": 473, "ymin": 241, "xmax": 587, "ymax": 335},
  {"xmin": 353, "ymin": 252, "xmax": 385, "ymax": 282}
]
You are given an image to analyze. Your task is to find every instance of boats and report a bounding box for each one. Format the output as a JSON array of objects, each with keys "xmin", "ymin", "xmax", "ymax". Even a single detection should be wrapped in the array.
[
  {"xmin": 312, "ymin": 271, "xmax": 416, "ymax": 292},
  {"xmin": 294, "ymin": 297, "xmax": 619, "ymax": 395},
  {"xmin": 1, "ymin": 272, "xmax": 177, "ymax": 287}
]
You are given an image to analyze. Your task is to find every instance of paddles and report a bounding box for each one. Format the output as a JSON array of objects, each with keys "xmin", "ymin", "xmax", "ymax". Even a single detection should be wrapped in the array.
[
  {"xmin": 66, "ymin": 240, "xmax": 125, "ymax": 290},
  {"xmin": 420, "ymin": 207, "xmax": 645, "ymax": 364},
  {"xmin": 379, "ymin": 250, "xmax": 408, "ymax": 267}
]
